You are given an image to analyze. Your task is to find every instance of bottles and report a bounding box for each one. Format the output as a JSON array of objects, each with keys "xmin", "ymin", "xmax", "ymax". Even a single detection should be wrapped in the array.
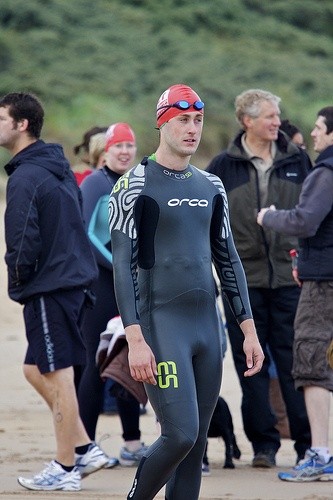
[{"xmin": 289, "ymin": 249, "xmax": 299, "ymax": 277}]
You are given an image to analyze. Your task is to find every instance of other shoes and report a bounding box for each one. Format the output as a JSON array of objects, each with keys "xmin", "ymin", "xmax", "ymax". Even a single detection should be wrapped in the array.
[
  {"xmin": 118, "ymin": 441, "xmax": 154, "ymax": 468},
  {"xmin": 103, "ymin": 452, "xmax": 119, "ymax": 470},
  {"xmin": 253, "ymin": 447, "xmax": 277, "ymax": 468}
]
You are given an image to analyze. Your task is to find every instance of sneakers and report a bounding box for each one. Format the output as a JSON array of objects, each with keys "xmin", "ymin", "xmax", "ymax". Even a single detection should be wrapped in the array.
[
  {"xmin": 279, "ymin": 448, "xmax": 333, "ymax": 481},
  {"xmin": 73, "ymin": 442, "xmax": 110, "ymax": 474},
  {"xmin": 16, "ymin": 459, "xmax": 82, "ymax": 492}
]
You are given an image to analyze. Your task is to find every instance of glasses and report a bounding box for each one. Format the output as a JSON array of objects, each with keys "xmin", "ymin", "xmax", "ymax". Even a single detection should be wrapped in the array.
[{"xmin": 156, "ymin": 99, "xmax": 205, "ymax": 111}]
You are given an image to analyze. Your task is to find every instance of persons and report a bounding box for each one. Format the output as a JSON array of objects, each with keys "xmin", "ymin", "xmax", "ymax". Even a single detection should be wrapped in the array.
[
  {"xmin": 257, "ymin": 106, "xmax": 333, "ymax": 482},
  {"xmin": 0, "ymin": 91, "xmax": 110, "ymax": 492},
  {"xmin": 70, "ymin": 121, "xmax": 308, "ymax": 475},
  {"xmin": 108, "ymin": 85, "xmax": 268, "ymax": 500},
  {"xmin": 202, "ymin": 90, "xmax": 311, "ymax": 468}
]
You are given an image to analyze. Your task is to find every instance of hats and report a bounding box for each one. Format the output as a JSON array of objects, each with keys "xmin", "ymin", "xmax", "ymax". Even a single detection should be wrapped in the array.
[
  {"xmin": 156, "ymin": 84, "xmax": 205, "ymax": 127},
  {"xmin": 104, "ymin": 123, "xmax": 136, "ymax": 152}
]
